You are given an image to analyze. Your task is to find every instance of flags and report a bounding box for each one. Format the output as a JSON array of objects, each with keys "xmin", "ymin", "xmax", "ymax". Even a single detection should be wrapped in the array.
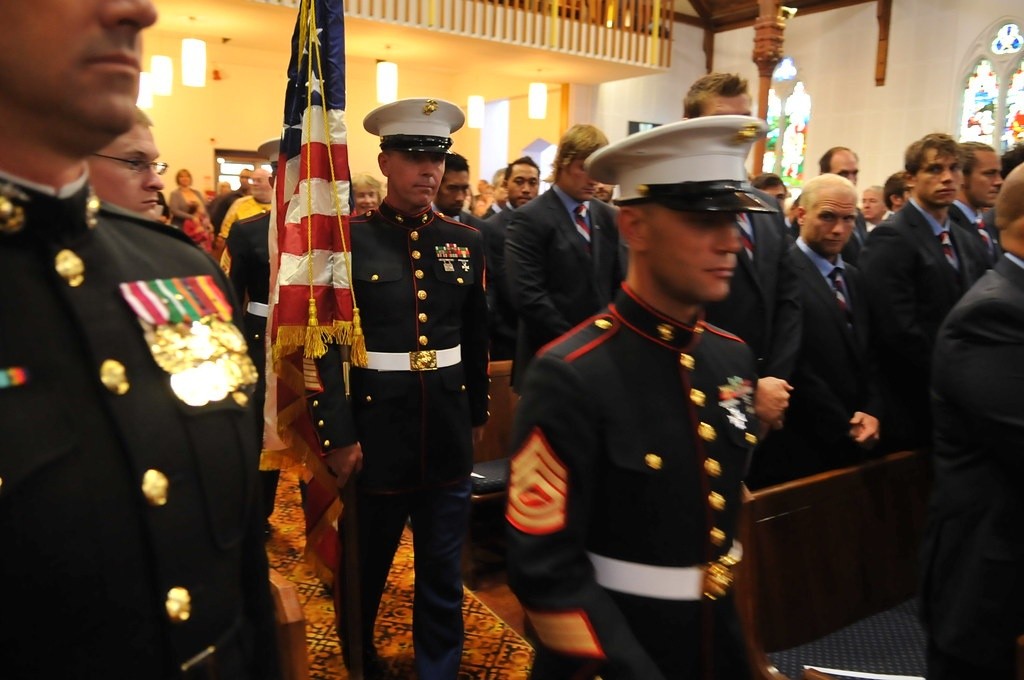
[{"xmin": 257, "ymin": 0, "xmax": 369, "ymax": 589}]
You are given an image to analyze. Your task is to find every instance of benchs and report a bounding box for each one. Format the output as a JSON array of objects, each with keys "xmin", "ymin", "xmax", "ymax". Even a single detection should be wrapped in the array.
[
  {"xmin": 732, "ymin": 449, "xmax": 930, "ymax": 680},
  {"xmin": 461, "ymin": 358, "xmax": 522, "ymax": 591}
]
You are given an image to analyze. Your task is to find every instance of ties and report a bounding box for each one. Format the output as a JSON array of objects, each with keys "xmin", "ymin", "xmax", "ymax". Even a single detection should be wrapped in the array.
[
  {"xmin": 832, "ymin": 270, "xmax": 851, "ymax": 328},
  {"xmin": 575, "ymin": 204, "xmax": 592, "ymax": 242},
  {"xmin": 940, "ymin": 229, "xmax": 958, "ymax": 270},
  {"xmin": 976, "ymin": 216, "xmax": 994, "ymax": 257}
]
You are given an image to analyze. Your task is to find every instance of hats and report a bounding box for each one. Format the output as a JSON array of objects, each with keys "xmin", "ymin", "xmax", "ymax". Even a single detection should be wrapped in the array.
[
  {"xmin": 584, "ymin": 115, "xmax": 778, "ymax": 215},
  {"xmin": 257, "ymin": 140, "xmax": 282, "ymax": 174},
  {"xmin": 362, "ymin": 98, "xmax": 465, "ymax": 156}
]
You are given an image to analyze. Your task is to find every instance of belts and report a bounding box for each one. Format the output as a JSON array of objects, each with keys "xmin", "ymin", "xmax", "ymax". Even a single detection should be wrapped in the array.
[
  {"xmin": 586, "ymin": 541, "xmax": 746, "ymax": 602},
  {"xmin": 354, "ymin": 344, "xmax": 462, "ymax": 371}
]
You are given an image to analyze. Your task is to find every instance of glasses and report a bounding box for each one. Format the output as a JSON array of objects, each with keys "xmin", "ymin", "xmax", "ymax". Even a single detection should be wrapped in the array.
[{"xmin": 93, "ymin": 150, "xmax": 168, "ymax": 176}]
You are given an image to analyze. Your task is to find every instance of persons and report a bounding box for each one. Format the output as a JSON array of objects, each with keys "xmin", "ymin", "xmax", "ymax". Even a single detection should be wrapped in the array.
[
  {"xmin": 80, "ymin": 105, "xmax": 169, "ymax": 213},
  {"xmin": 502, "ymin": 114, "xmax": 768, "ymax": 680},
  {"xmin": 863, "ymin": 133, "xmax": 989, "ymax": 452},
  {"xmin": 170, "ymin": 72, "xmax": 1024, "ymax": 492},
  {"xmin": 914, "ymin": 162, "xmax": 1024, "ymax": 680},
  {"xmin": 0, "ymin": 0, "xmax": 285, "ymax": 680},
  {"xmin": 301, "ymin": 98, "xmax": 492, "ymax": 680}
]
